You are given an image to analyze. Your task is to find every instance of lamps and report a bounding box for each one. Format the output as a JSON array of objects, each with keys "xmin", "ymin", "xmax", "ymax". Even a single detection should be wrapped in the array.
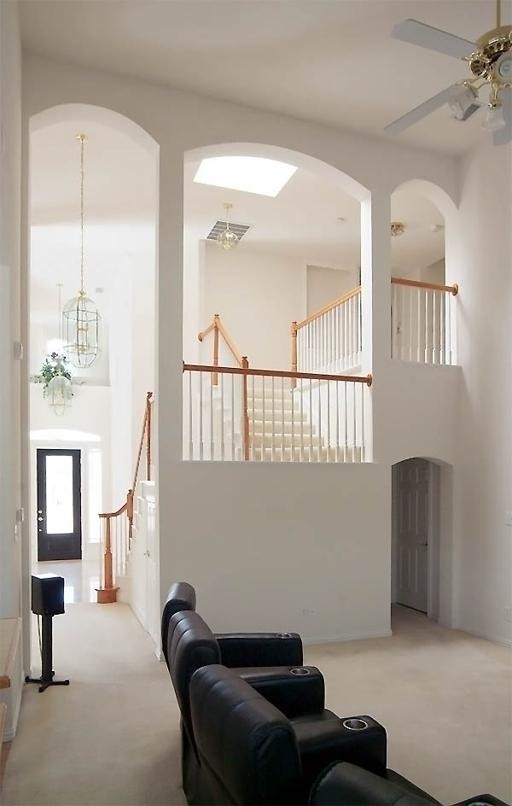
[
  {"xmin": 392, "ymin": 222, "xmax": 404, "ymax": 237},
  {"xmin": 61, "ymin": 135, "xmax": 101, "ymax": 369},
  {"xmin": 218, "ymin": 202, "xmax": 239, "ymax": 249},
  {"xmin": 46, "ymin": 284, "xmax": 71, "ymax": 415},
  {"xmin": 446, "ymin": 80, "xmax": 506, "ymax": 132},
  {"xmin": 25, "ymin": 573, "xmax": 70, "ymax": 693}
]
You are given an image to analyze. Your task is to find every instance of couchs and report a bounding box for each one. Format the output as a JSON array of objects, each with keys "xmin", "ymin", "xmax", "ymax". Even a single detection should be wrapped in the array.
[
  {"xmin": 168, "ymin": 615, "xmax": 343, "ymax": 800},
  {"xmin": 162, "ymin": 582, "xmax": 305, "ymax": 747},
  {"xmin": 190, "ymin": 665, "xmax": 443, "ymax": 805},
  {"xmin": 306, "ymin": 762, "xmax": 511, "ymax": 806}
]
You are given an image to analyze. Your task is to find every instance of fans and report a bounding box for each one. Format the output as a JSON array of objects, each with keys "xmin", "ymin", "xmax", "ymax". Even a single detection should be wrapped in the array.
[{"xmin": 383, "ymin": 0, "xmax": 512, "ymax": 136}]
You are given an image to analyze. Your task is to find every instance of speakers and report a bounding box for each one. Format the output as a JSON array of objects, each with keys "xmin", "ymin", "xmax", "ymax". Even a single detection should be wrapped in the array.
[{"xmin": 31, "ymin": 575, "xmax": 65, "ymax": 616}]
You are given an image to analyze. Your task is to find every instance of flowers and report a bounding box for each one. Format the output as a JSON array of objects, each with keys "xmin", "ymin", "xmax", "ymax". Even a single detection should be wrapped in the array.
[{"xmin": 30, "ymin": 352, "xmax": 71, "ymax": 397}]
